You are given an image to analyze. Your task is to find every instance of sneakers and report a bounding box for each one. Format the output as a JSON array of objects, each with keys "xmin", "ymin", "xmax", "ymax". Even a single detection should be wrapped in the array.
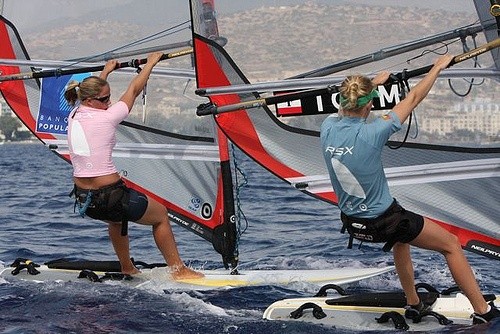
[
  {"xmin": 405, "ymin": 300, "xmax": 430, "ymax": 319},
  {"xmin": 473, "ymin": 302, "xmax": 500, "ymax": 325}
]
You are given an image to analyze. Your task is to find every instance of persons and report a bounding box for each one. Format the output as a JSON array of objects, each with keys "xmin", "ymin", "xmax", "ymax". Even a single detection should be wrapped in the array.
[
  {"xmin": 320, "ymin": 53, "xmax": 500, "ymax": 324},
  {"xmin": 65, "ymin": 52, "xmax": 204, "ymax": 281}
]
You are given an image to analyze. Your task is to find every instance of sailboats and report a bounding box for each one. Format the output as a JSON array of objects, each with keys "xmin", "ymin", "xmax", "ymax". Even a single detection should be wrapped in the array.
[
  {"xmin": 0, "ymin": 1, "xmax": 396, "ymax": 291},
  {"xmin": 193, "ymin": 1, "xmax": 500, "ymax": 331}
]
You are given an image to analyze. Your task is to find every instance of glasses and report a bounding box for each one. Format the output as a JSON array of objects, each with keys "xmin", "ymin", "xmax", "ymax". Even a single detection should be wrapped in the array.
[{"xmin": 92, "ymin": 94, "xmax": 111, "ymax": 103}]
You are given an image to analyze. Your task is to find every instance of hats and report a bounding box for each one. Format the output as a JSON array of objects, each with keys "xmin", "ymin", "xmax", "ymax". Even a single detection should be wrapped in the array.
[{"xmin": 340, "ymin": 88, "xmax": 380, "ymax": 108}]
